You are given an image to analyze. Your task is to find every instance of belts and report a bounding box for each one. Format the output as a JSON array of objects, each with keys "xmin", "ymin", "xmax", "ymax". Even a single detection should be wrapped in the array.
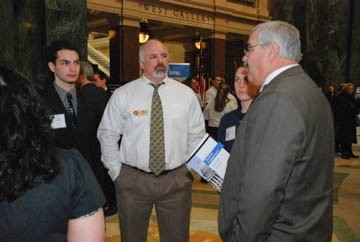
[{"xmin": 121, "ymin": 162, "xmax": 185, "ymax": 177}]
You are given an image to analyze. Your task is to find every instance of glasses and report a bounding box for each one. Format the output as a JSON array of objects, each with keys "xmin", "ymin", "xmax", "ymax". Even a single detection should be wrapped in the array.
[{"xmin": 243, "ymin": 41, "xmax": 271, "ymax": 53}]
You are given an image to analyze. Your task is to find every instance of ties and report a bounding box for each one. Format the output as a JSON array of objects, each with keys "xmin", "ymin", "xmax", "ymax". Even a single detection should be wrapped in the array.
[
  {"xmin": 65, "ymin": 93, "xmax": 76, "ymax": 126},
  {"xmin": 149, "ymin": 82, "xmax": 165, "ymax": 177}
]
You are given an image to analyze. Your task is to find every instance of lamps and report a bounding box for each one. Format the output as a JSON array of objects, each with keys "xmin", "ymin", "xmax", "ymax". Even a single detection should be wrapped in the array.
[
  {"xmin": 193, "ymin": 37, "xmax": 200, "ymax": 49},
  {"xmin": 139, "ymin": 21, "xmax": 150, "ymax": 44}
]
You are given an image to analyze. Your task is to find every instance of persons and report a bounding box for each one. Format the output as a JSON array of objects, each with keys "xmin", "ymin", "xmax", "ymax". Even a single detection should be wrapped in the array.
[
  {"xmin": 97, "ymin": 39, "xmax": 206, "ymax": 242},
  {"xmin": 37, "ymin": 40, "xmax": 117, "ymax": 217},
  {"xmin": 182, "ymin": 76, "xmax": 239, "ymax": 183},
  {"xmin": 217, "ymin": 61, "xmax": 261, "ymax": 154},
  {"xmin": 218, "ymin": 21, "xmax": 335, "ymax": 242},
  {"xmin": 319, "ymin": 83, "xmax": 360, "ymax": 160},
  {"xmin": 0, "ymin": 65, "xmax": 107, "ymax": 242}
]
